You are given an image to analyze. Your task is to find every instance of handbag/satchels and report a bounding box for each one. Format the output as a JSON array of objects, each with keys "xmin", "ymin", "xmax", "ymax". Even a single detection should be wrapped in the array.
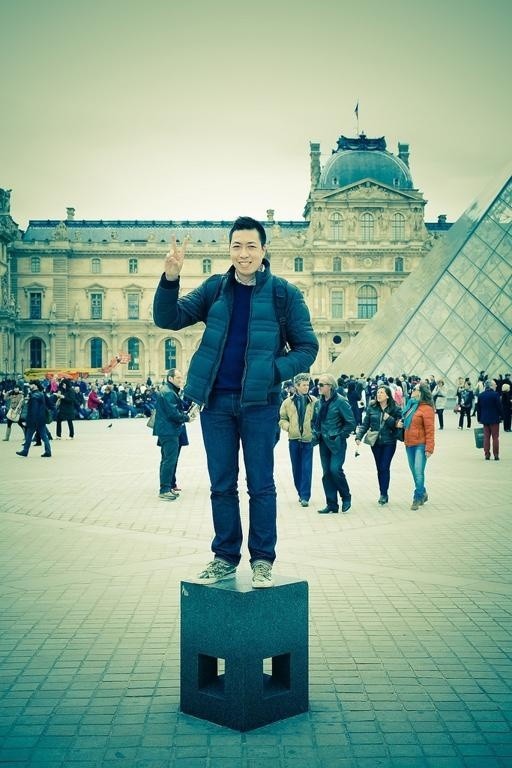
[
  {"xmin": 147, "ymin": 408, "xmax": 156, "ymax": 428},
  {"xmin": 45, "ymin": 408, "xmax": 52, "ymax": 423},
  {"xmin": 6, "ymin": 407, "xmax": 19, "ymax": 421},
  {"xmin": 474, "ymin": 428, "xmax": 484, "ymax": 447},
  {"xmin": 454, "ymin": 404, "xmax": 462, "ymax": 412},
  {"xmin": 363, "ymin": 430, "xmax": 380, "ymax": 446}
]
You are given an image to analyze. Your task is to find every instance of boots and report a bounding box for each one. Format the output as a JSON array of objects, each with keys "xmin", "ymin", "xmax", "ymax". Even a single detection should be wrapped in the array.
[
  {"xmin": 2, "ymin": 427, "xmax": 11, "ymax": 441},
  {"xmin": 22, "ymin": 426, "xmax": 26, "ymax": 439}
]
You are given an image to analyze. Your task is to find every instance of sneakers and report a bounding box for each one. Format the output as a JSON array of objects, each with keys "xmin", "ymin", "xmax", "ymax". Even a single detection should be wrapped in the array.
[
  {"xmin": 250, "ymin": 559, "xmax": 272, "ymax": 587},
  {"xmin": 159, "ymin": 491, "xmax": 176, "ymax": 499},
  {"xmin": 66, "ymin": 436, "xmax": 74, "ymax": 439},
  {"xmin": 465, "ymin": 427, "xmax": 471, "ymax": 429},
  {"xmin": 52, "ymin": 435, "xmax": 61, "ymax": 439},
  {"xmin": 174, "ymin": 487, "xmax": 181, "ymax": 490},
  {"xmin": 301, "ymin": 500, "xmax": 308, "ymax": 506},
  {"xmin": 170, "ymin": 488, "xmax": 179, "ymax": 496},
  {"xmin": 423, "ymin": 492, "xmax": 428, "ymax": 501},
  {"xmin": 411, "ymin": 497, "xmax": 425, "ymax": 509},
  {"xmin": 193, "ymin": 556, "xmax": 236, "ymax": 584},
  {"xmin": 457, "ymin": 426, "xmax": 463, "ymax": 430}
]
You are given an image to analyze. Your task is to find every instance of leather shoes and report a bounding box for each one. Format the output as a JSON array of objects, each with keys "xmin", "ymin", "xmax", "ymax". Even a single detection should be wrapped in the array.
[
  {"xmin": 485, "ymin": 456, "xmax": 490, "ymax": 459},
  {"xmin": 378, "ymin": 494, "xmax": 389, "ymax": 505},
  {"xmin": 16, "ymin": 449, "xmax": 28, "ymax": 456},
  {"xmin": 41, "ymin": 452, "xmax": 51, "ymax": 457},
  {"xmin": 342, "ymin": 493, "xmax": 351, "ymax": 511},
  {"xmin": 33, "ymin": 441, "xmax": 42, "ymax": 446},
  {"xmin": 318, "ymin": 502, "xmax": 338, "ymax": 513},
  {"xmin": 495, "ymin": 457, "xmax": 499, "ymax": 460}
]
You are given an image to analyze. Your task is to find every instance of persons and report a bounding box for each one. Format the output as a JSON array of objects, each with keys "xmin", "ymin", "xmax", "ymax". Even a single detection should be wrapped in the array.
[
  {"xmin": 279, "ymin": 374, "xmax": 319, "ymax": 506},
  {"xmin": 457, "ymin": 371, "xmax": 488, "ymax": 429},
  {"xmin": 396, "ymin": 382, "xmax": 434, "ymax": 510},
  {"xmin": 495, "ymin": 373, "xmax": 512, "ymax": 432},
  {"xmin": 279, "ymin": 378, "xmax": 319, "ymax": 404},
  {"xmin": 0, "ymin": 373, "xmax": 166, "ymax": 458},
  {"xmin": 476, "ymin": 379, "xmax": 505, "ymax": 460},
  {"xmin": 151, "ymin": 391, "xmax": 192, "ymax": 491},
  {"xmin": 153, "ymin": 217, "xmax": 319, "ymax": 591},
  {"xmin": 432, "ymin": 380, "xmax": 446, "ymax": 430},
  {"xmin": 310, "ymin": 374, "xmax": 356, "ymax": 513},
  {"xmin": 356, "ymin": 384, "xmax": 405, "ymax": 504},
  {"xmin": 368, "ymin": 374, "xmax": 436, "ymax": 404},
  {"xmin": 149, "ymin": 367, "xmax": 195, "ymax": 499},
  {"xmin": 337, "ymin": 374, "xmax": 367, "ymax": 433}
]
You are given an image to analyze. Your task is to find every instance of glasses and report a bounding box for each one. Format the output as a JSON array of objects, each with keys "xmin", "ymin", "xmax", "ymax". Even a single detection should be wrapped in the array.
[
  {"xmin": 411, "ymin": 386, "xmax": 420, "ymax": 391},
  {"xmin": 315, "ymin": 382, "xmax": 332, "ymax": 388},
  {"xmin": 491, "ymin": 383, "xmax": 497, "ymax": 385}
]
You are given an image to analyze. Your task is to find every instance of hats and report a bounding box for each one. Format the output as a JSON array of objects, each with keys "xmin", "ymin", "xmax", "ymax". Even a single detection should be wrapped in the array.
[{"xmin": 28, "ymin": 379, "xmax": 39, "ymax": 384}]
